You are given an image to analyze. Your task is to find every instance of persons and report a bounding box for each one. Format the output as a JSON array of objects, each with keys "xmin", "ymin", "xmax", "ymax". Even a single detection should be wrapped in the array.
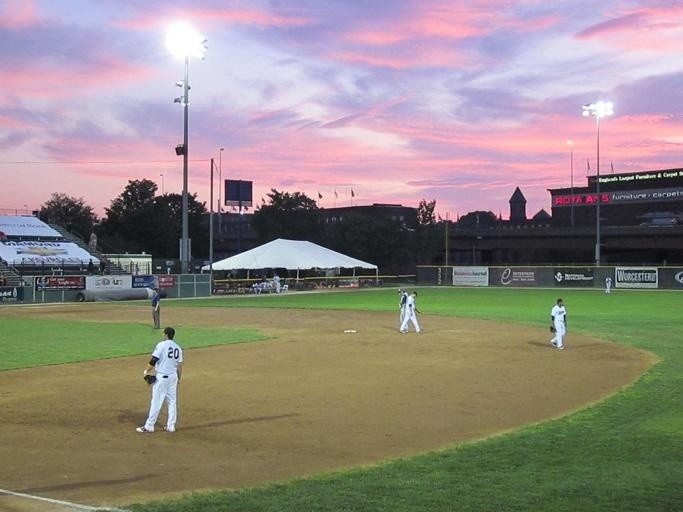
[
  {"xmin": 252, "ymin": 278, "xmax": 262, "ymax": 294},
  {"xmin": 398, "ymin": 288, "xmax": 409, "ymax": 333},
  {"xmin": 272, "ymin": 271, "xmax": 280, "ymax": 294},
  {"xmin": 549, "ymin": 298, "xmax": 567, "ymax": 350},
  {"xmin": 399, "ymin": 292, "xmax": 423, "ymax": 334},
  {"xmin": 135, "ymin": 327, "xmax": 182, "ymax": 432},
  {"xmin": 97, "ymin": 258, "xmax": 106, "ymax": 276},
  {"xmin": 604, "ymin": 273, "xmax": 613, "ymax": 294},
  {"xmin": 86, "ymin": 258, "xmax": 93, "ymax": 273},
  {"xmin": 151, "ymin": 287, "xmax": 159, "ymax": 328}
]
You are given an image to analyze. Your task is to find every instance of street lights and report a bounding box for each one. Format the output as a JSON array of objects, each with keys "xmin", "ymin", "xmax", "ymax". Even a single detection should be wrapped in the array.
[
  {"xmin": 166, "ymin": 32, "xmax": 224, "ymax": 274},
  {"xmin": 582, "ymin": 101, "xmax": 613, "ymax": 265}
]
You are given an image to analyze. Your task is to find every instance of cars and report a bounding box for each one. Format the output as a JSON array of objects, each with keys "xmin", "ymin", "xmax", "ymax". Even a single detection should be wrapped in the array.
[{"xmin": 647, "ymin": 217, "xmax": 677, "ymax": 226}]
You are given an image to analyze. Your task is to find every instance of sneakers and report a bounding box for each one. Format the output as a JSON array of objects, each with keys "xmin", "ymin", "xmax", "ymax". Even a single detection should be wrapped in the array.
[
  {"xmin": 135, "ymin": 426, "xmax": 151, "ymax": 432},
  {"xmin": 550, "ymin": 339, "xmax": 565, "ymax": 350}
]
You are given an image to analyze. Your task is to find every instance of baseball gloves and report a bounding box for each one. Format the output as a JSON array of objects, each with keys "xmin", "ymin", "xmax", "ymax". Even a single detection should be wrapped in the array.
[
  {"xmin": 550, "ymin": 325, "xmax": 556, "ymax": 333},
  {"xmin": 144, "ymin": 375, "xmax": 157, "ymax": 386}
]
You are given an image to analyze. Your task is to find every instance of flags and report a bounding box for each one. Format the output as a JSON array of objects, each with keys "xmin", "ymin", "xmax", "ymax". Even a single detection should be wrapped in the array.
[
  {"xmin": 220, "ymin": 207, "xmax": 223, "ymax": 212},
  {"xmin": 244, "ymin": 206, "xmax": 248, "ymax": 211},
  {"xmin": 587, "ymin": 161, "xmax": 590, "ymax": 170},
  {"xmin": 318, "ymin": 193, "xmax": 322, "ymax": 200},
  {"xmin": 232, "ymin": 206, "xmax": 236, "ymax": 211},
  {"xmin": 610, "ymin": 162, "xmax": 614, "ymax": 173},
  {"xmin": 335, "ymin": 191, "xmax": 337, "ymax": 198},
  {"xmin": 351, "ymin": 188, "xmax": 353, "ymax": 197}
]
